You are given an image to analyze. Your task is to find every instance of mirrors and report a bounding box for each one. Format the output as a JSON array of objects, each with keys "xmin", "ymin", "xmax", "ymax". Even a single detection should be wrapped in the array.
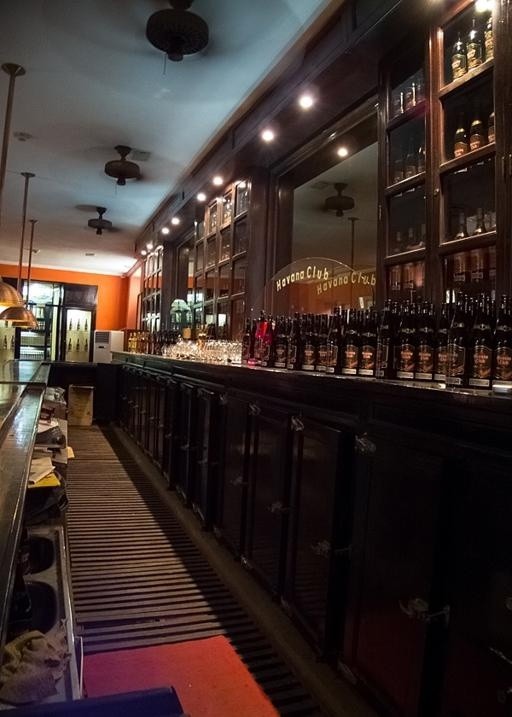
[{"xmin": 265, "ymin": 91, "xmax": 383, "ymax": 326}]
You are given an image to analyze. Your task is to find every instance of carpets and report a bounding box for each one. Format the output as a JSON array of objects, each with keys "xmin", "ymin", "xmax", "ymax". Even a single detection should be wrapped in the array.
[{"xmin": 79, "ymin": 635, "xmax": 281, "ymax": 717}]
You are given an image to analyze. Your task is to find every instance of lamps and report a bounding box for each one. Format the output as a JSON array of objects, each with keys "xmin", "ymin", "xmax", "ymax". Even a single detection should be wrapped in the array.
[
  {"xmin": 88, "ymin": 0, "xmax": 207, "ymax": 234},
  {"xmin": 324, "ymin": 182, "xmax": 355, "ymax": 218},
  {"xmin": 0, "ymin": 58, "xmax": 40, "ymax": 331}
]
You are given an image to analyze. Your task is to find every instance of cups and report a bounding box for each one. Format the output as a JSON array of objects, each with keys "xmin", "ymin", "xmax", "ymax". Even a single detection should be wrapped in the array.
[{"xmin": 160, "ymin": 339, "xmax": 242, "ymax": 365}]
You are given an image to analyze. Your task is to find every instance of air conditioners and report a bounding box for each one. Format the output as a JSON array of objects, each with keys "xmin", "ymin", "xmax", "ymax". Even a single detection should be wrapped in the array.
[{"xmin": 93, "ymin": 330, "xmax": 111, "ymax": 364}]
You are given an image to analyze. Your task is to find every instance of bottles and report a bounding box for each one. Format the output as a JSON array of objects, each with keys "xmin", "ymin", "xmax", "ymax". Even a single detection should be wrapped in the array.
[
  {"xmin": 389, "ymin": 68, "xmax": 427, "ymax": 120},
  {"xmin": 391, "ymin": 131, "xmax": 426, "ymax": 197},
  {"xmin": 450, "ymin": 205, "xmax": 496, "ymax": 289},
  {"xmin": 69, "ymin": 318, "xmax": 88, "ymax": 331},
  {"xmin": 241, "ymin": 290, "xmax": 512, "ymax": 392},
  {"xmin": 68, "ymin": 338, "xmax": 88, "ymax": 352},
  {"xmin": 127, "ymin": 329, "xmax": 182, "ymax": 357},
  {"xmin": 388, "ymin": 222, "xmax": 426, "ymax": 294},
  {"xmin": 451, "ymin": 11, "xmax": 495, "ymax": 81},
  {"xmin": 452, "ymin": 105, "xmax": 495, "ymax": 175},
  {"xmin": 2, "ymin": 320, "xmax": 16, "ymax": 350}
]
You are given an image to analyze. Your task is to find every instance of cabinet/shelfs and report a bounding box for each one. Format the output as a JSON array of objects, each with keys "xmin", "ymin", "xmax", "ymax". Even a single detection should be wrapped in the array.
[
  {"xmin": 382, "ymin": 0, "xmax": 512, "ymax": 320},
  {"xmin": 139, "ymin": 173, "xmax": 251, "ymax": 343}
]
